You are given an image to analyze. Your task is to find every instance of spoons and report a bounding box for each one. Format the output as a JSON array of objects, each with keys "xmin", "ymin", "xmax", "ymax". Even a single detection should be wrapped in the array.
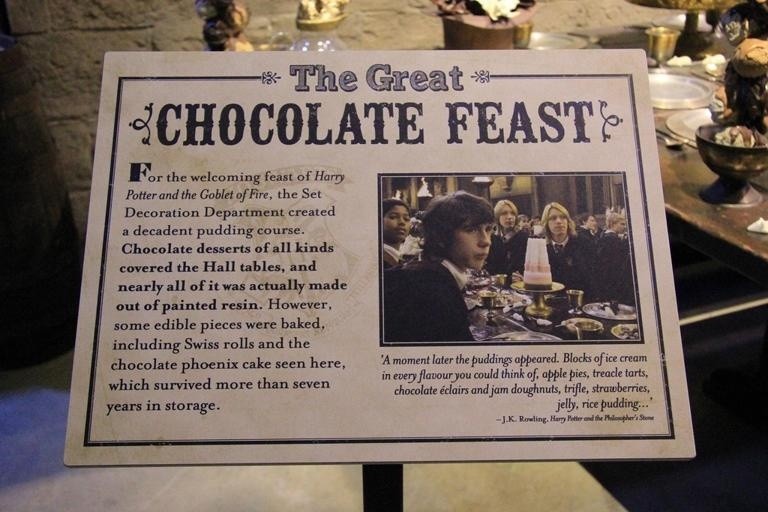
[{"xmin": 655, "ymin": 132, "xmax": 683, "ymax": 149}]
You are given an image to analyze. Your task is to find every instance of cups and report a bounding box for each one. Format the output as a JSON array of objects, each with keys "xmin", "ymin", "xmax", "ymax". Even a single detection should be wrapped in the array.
[{"xmin": 574, "ymin": 319, "xmax": 603, "ymax": 341}]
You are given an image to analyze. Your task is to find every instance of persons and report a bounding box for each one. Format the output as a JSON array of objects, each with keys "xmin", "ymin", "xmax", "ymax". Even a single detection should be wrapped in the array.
[{"xmin": 384, "ymin": 191, "xmax": 635, "ymax": 343}]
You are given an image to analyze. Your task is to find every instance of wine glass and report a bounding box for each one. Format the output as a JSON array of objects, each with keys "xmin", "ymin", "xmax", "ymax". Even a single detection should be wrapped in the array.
[
  {"xmin": 480, "ymin": 292, "xmax": 497, "ymax": 317},
  {"xmin": 643, "ymin": 25, "xmax": 682, "ymax": 69},
  {"xmin": 565, "ymin": 289, "xmax": 584, "ymax": 315},
  {"xmin": 694, "ymin": 122, "xmax": 768, "ymax": 210}
]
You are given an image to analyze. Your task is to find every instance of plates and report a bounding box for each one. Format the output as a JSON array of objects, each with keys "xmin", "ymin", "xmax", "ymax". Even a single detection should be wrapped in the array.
[
  {"xmin": 582, "ymin": 301, "xmax": 637, "ymax": 321},
  {"xmin": 647, "ymin": 71, "xmax": 715, "ymax": 110},
  {"xmin": 492, "ymin": 331, "xmax": 565, "ymax": 341},
  {"xmin": 664, "ymin": 108, "xmax": 716, "ymax": 142},
  {"xmin": 610, "ymin": 323, "xmax": 639, "ymax": 341}
]
